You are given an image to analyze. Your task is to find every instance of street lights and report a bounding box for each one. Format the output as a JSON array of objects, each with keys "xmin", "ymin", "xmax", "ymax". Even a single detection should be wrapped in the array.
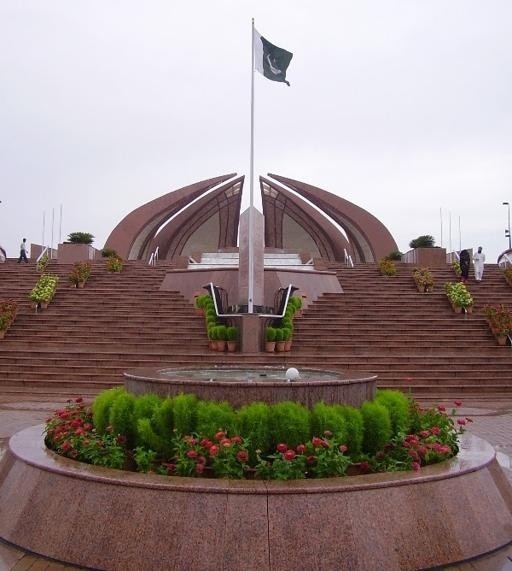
[{"xmin": 501, "ymin": 201, "xmax": 511, "ymax": 249}]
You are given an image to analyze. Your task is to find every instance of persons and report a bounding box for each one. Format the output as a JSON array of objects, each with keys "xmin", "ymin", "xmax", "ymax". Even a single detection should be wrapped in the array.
[
  {"xmin": 472, "ymin": 246, "xmax": 486, "ymax": 281},
  {"xmin": 459, "ymin": 249, "xmax": 471, "ymax": 283},
  {"xmin": 16, "ymin": 238, "xmax": 29, "ymax": 263}
]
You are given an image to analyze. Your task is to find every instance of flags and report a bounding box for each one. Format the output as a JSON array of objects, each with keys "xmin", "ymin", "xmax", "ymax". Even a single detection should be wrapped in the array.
[{"xmin": 253, "ymin": 27, "xmax": 294, "ymax": 87}]
